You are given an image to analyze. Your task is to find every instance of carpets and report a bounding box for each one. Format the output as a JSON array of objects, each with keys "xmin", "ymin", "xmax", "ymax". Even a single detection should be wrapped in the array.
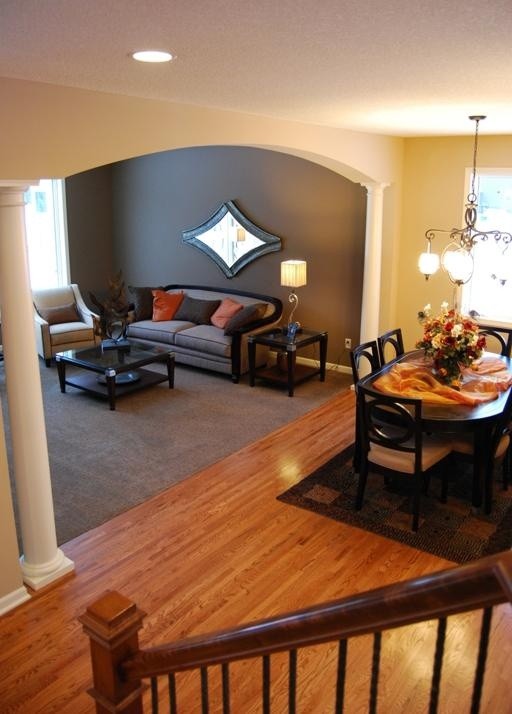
[
  {"xmin": 277, "ymin": 443, "xmax": 512, "ymax": 565},
  {"xmin": 0, "ymin": 356, "xmax": 354, "ymax": 555}
]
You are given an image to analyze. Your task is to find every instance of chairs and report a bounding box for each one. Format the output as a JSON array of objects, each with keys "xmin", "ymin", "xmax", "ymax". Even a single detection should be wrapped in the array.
[
  {"xmin": 349, "ymin": 340, "xmax": 382, "ymax": 474},
  {"xmin": 33, "ymin": 284, "xmax": 100, "ymax": 368},
  {"xmin": 378, "ymin": 328, "xmax": 404, "ymax": 367},
  {"xmin": 453, "ymin": 387, "xmax": 512, "ymax": 515},
  {"xmin": 479, "ymin": 324, "xmax": 512, "ymax": 358},
  {"xmin": 355, "ymin": 384, "xmax": 454, "ymax": 532}
]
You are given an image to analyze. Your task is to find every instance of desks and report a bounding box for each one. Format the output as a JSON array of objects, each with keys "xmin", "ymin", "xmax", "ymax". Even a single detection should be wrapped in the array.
[{"xmin": 358, "ymin": 349, "xmax": 512, "ymax": 506}]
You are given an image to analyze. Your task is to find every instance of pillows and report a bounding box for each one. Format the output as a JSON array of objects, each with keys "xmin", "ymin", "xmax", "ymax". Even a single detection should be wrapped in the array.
[
  {"xmin": 37, "ymin": 303, "xmax": 81, "ymax": 325},
  {"xmin": 128, "ymin": 286, "xmax": 268, "ymax": 337}
]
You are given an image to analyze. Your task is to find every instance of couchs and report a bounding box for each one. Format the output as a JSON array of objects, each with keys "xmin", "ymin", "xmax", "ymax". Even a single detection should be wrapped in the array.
[{"xmin": 126, "ymin": 286, "xmax": 283, "ymax": 384}]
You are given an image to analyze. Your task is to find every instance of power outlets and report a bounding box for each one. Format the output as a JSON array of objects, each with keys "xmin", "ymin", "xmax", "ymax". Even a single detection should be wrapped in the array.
[{"xmin": 345, "ymin": 338, "xmax": 352, "ymax": 349}]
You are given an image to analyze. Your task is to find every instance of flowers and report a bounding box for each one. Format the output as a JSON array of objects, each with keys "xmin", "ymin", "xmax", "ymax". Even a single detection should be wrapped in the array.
[{"xmin": 415, "ymin": 301, "xmax": 487, "ymax": 391}]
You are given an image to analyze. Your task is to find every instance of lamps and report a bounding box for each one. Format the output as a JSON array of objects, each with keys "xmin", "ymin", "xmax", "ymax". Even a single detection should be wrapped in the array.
[
  {"xmin": 418, "ymin": 116, "xmax": 512, "ymax": 286},
  {"xmin": 280, "ymin": 260, "xmax": 307, "ymax": 331}
]
